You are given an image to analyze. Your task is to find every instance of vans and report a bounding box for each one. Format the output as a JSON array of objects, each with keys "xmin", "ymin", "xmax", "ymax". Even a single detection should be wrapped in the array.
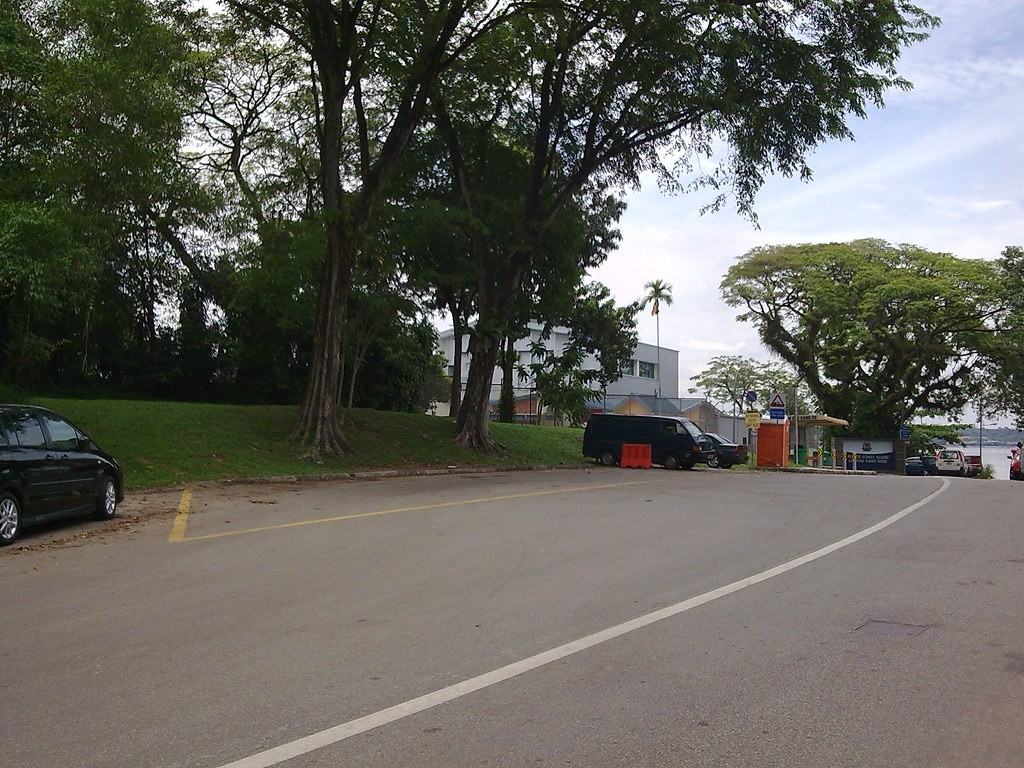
[
  {"xmin": 963, "ymin": 455, "xmax": 984, "ymax": 478},
  {"xmin": 582, "ymin": 412, "xmax": 717, "ymax": 470},
  {"xmin": 934, "ymin": 448, "xmax": 969, "ymax": 477}
]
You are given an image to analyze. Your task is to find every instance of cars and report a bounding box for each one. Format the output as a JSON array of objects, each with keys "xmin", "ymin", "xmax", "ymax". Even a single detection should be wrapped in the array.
[
  {"xmin": 697, "ymin": 432, "xmax": 749, "ymax": 469},
  {"xmin": 0, "ymin": 403, "xmax": 124, "ymax": 546},
  {"xmin": 1007, "ymin": 439, "xmax": 1024, "ymax": 481},
  {"xmin": 904, "ymin": 455, "xmax": 938, "ymax": 477}
]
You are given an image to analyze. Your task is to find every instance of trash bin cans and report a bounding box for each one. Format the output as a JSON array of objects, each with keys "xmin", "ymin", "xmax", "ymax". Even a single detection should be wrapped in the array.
[
  {"xmin": 822, "ymin": 451, "xmax": 831, "ymax": 465},
  {"xmin": 794, "ymin": 443, "xmax": 807, "ymax": 465},
  {"xmin": 922, "ymin": 456, "xmax": 937, "ymax": 476}
]
